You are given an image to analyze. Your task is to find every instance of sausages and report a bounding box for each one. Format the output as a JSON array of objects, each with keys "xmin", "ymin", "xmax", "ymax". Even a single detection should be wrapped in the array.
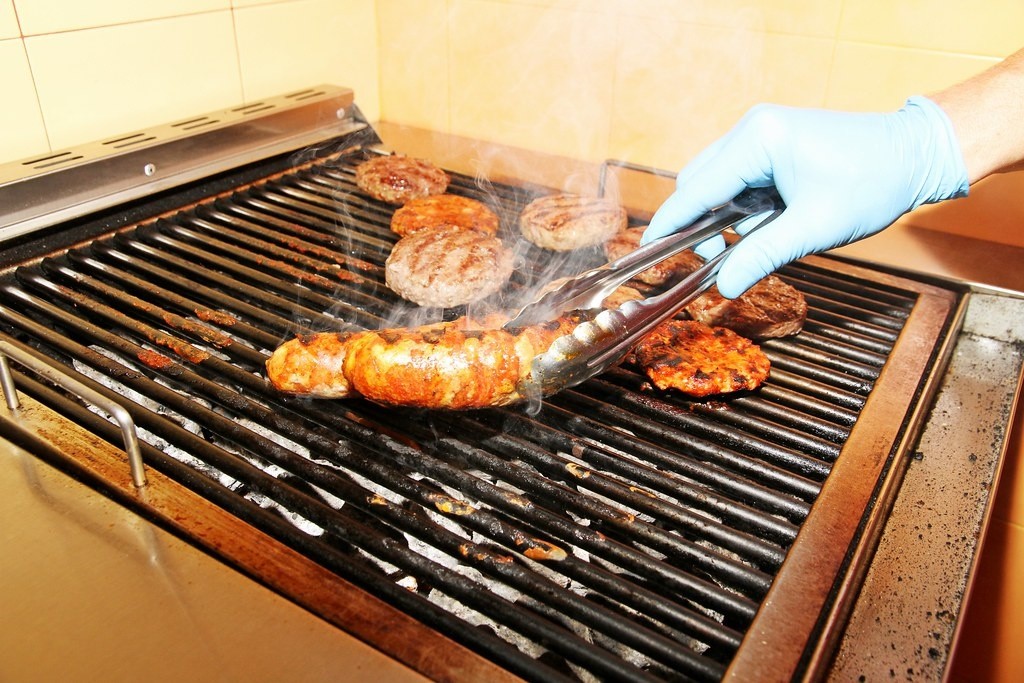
[
  {"xmin": 343, "ymin": 307, "xmax": 634, "ymax": 408},
  {"xmin": 265, "ymin": 308, "xmax": 529, "ymax": 400}
]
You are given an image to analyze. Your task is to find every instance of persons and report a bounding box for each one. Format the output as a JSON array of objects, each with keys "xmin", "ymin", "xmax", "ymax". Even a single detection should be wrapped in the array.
[{"xmin": 641, "ymin": 44, "xmax": 1023, "ymax": 301}]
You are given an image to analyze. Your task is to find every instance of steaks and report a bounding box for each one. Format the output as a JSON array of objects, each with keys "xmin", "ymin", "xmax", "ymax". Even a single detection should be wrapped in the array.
[
  {"xmin": 533, "ymin": 276, "xmax": 646, "ymax": 310},
  {"xmin": 383, "ymin": 223, "xmax": 515, "ymax": 308},
  {"xmin": 356, "ymin": 154, "xmax": 449, "ymax": 206},
  {"xmin": 603, "ymin": 225, "xmax": 710, "ymax": 286},
  {"xmin": 684, "ymin": 273, "xmax": 811, "ymax": 339},
  {"xmin": 390, "ymin": 194, "xmax": 500, "ymax": 235},
  {"xmin": 518, "ymin": 192, "xmax": 628, "ymax": 251},
  {"xmin": 634, "ymin": 319, "xmax": 771, "ymax": 396}
]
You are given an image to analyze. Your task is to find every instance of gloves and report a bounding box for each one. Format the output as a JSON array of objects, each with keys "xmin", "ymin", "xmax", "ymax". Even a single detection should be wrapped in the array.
[{"xmin": 640, "ymin": 96, "xmax": 969, "ymax": 299}]
